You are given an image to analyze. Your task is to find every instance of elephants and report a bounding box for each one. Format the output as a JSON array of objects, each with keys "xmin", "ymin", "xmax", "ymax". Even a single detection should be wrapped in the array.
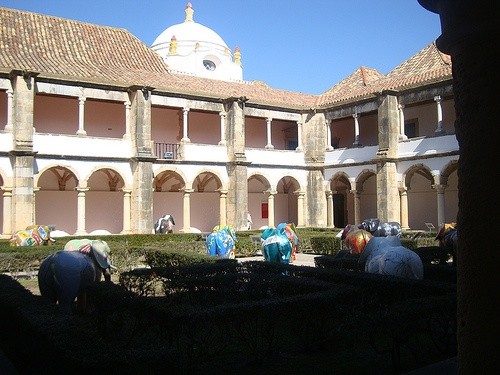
[
  {"xmin": 38, "ymin": 250, "xmax": 96, "ymax": 306},
  {"xmin": 154, "ymin": 214, "xmax": 175, "ymax": 234},
  {"xmin": 25, "ymin": 225, "xmax": 56, "ymax": 246},
  {"xmin": 212, "ymin": 224, "xmax": 236, "ymax": 233},
  {"xmin": 434, "ymin": 224, "xmax": 457, "ymax": 265},
  {"xmin": 340, "ymin": 224, "xmax": 373, "ymax": 254},
  {"xmin": 260, "ymin": 227, "xmax": 298, "ymax": 264},
  {"xmin": 205, "ymin": 226, "xmax": 237, "ymax": 259},
  {"xmin": 276, "ymin": 222, "xmax": 299, "ymax": 263},
  {"xmin": 63, "ymin": 239, "xmax": 118, "ymax": 282},
  {"xmin": 247, "ymin": 213, "xmax": 253, "ymax": 230},
  {"xmin": 357, "ymin": 234, "xmax": 424, "ymax": 280},
  {"xmin": 358, "ymin": 218, "xmax": 402, "ymax": 238},
  {"xmin": 9, "ymin": 226, "xmax": 49, "ymax": 247}
]
